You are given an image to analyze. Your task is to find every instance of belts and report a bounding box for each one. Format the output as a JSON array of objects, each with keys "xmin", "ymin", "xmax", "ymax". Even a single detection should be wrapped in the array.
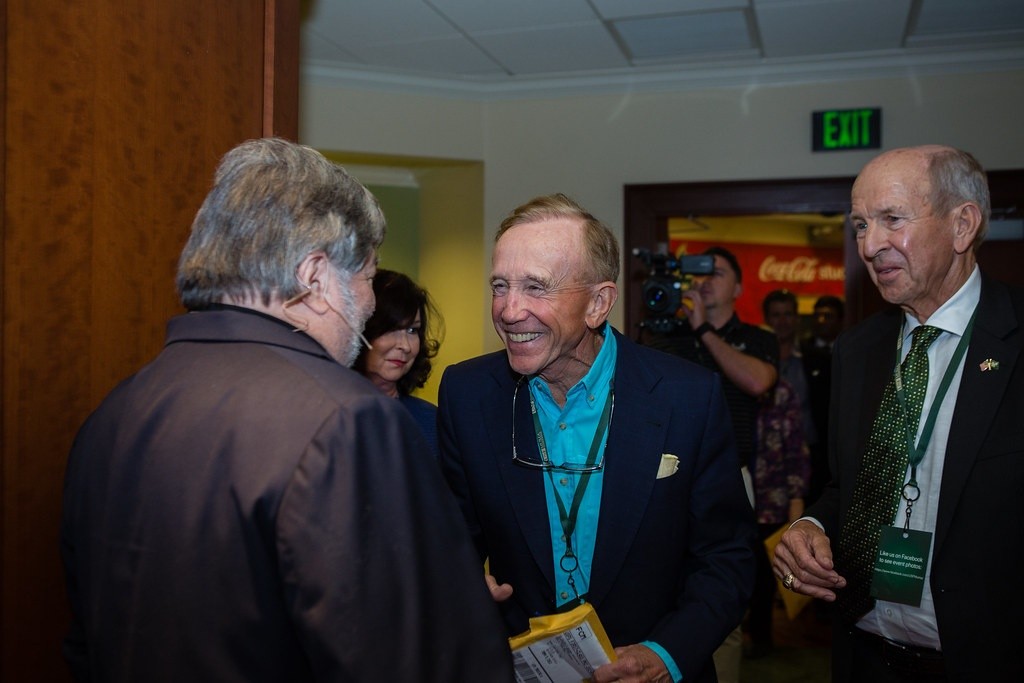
[{"xmin": 849, "ymin": 628, "xmax": 944, "ymax": 679}]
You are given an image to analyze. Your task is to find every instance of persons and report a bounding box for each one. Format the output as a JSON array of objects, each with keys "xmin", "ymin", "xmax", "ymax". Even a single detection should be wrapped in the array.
[
  {"xmin": 771, "ymin": 144, "xmax": 1024, "ymax": 683},
  {"xmin": 639, "ymin": 248, "xmax": 845, "ymax": 660},
  {"xmin": 61, "ymin": 138, "xmax": 522, "ymax": 683},
  {"xmin": 440, "ymin": 191, "xmax": 779, "ymax": 683},
  {"xmin": 352, "ymin": 269, "xmax": 440, "ymax": 465}
]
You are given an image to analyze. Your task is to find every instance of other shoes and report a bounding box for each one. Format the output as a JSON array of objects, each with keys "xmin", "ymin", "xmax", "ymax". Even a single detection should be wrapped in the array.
[{"xmin": 743, "ymin": 630, "xmax": 773, "ymax": 658}]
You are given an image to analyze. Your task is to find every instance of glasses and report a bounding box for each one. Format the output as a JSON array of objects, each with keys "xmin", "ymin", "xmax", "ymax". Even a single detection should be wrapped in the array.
[{"xmin": 510, "ymin": 387, "xmax": 615, "ymax": 471}]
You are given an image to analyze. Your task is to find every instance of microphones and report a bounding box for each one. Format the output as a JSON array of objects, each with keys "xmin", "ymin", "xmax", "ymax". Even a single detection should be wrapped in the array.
[{"xmin": 324, "ymin": 298, "xmax": 373, "ymax": 351}]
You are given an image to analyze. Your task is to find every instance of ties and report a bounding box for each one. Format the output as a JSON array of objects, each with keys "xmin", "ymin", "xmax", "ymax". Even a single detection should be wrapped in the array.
[{"xmin": 831, "ymin": 325, "xmax": 942, "ymax": 613}]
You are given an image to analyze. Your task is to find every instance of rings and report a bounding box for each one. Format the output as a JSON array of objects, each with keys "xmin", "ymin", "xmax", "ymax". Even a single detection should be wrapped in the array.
[{"xmin": 783, "ymin": 573, "xmax": 794, "ymax": 589}]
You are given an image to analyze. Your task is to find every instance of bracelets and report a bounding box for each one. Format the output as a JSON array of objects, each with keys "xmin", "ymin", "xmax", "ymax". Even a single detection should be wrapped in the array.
[{"xmin": 696, "ymin": 321, "xmax": 721, "ymax": 343}]
